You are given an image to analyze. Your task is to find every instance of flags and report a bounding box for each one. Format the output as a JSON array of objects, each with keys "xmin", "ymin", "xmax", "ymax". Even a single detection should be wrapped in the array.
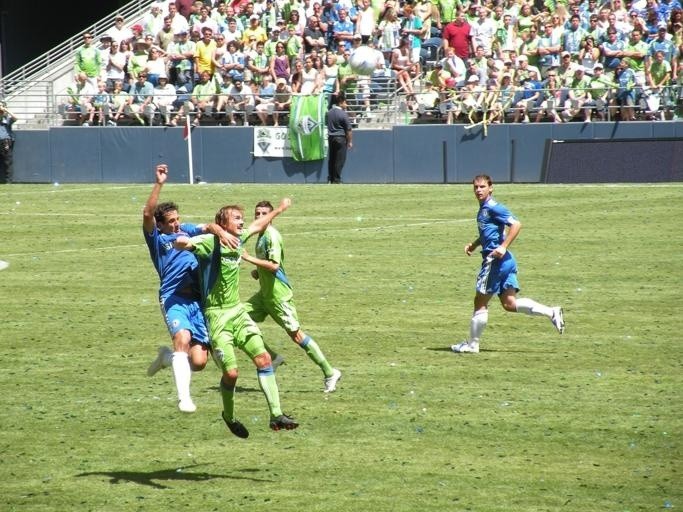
[{"xmin": 289, "ymin": 94, "xmax": 326, "ymax": 162}]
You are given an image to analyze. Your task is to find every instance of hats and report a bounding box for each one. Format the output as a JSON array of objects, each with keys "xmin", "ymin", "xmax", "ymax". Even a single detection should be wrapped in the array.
[
  {"xmin": 593, "ymin": 62, "xmax": 603, "ymax": 69},
  {"xmin": 657, "ymin": 22, "xmax": 668, "ymax": 30},
  {"xmin": 517, "ymin": 55, "xmax": 526, "ymax": 61},
  {"xmin": 157, "ymin": 73, "xmax": 169, "ymax": 83},
  {"xmin": 575, "ymin": 66, "xmax": 583, "ymax": 72},
  {"xmin": 130, "ymin": 24, "xmax": 143, "ymax": 31},
  {"xmin": 467, "ymin": 75, "xmax": 478, "ymax": 82},
  {"xmin": 133, "ymin": 39, "xmax": 149, "ymax": 49},
  {"xmin": 276, "ymin": 78, "xmax": 285, "ymax": 85},
  {"xmin": 100, "ymin": 34, "xmax": 112, "ymax": 41},
  {"xmin": 560, "ymin": 51, "xmax": 569, "ymax": 58}
]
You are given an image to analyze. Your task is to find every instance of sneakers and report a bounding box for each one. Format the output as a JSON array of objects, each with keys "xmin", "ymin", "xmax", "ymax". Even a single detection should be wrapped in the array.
[
  {"xmin": 178, "ymin": 398, "xmax": 196, "ymax": 413},
  {"xmin": 272, "ymin": 355, "xmax": 283, "ymax": 371},
  {"xmin": 222, "ymin": 411, "xmax": 249, "ymax": 438},
  {"xmin": 324, "ymin": 370, "xmax": 341, "ymax": 393},
  {"xmin": 451, "ymin": 341, "xmax": 479, "ymax": 353},
  {"xmin": 148, "ymin": 347, "xmax": 168, "ymax": 375},
  {"xmin": 270, "ymin": 413, "xmax": 298, "ymax": 431},
  {"xmin": 552, "ymin": 306, "xmax": 565, "ymax": 334}
]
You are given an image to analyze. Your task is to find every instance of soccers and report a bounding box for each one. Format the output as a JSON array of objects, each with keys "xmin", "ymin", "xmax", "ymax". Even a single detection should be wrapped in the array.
[{"xmin": 349, "ymin": 46, "xmax": 378, "ymax": 74}]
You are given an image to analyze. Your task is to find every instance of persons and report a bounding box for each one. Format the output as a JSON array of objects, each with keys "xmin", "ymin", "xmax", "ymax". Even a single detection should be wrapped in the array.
[
  {"xmin": 172, "ymin": 194, "xmax": 301, "ymax": 438},
  {"xmin": 243, "ymin": 198, "xmax": 342, "ymax": 394},
  {"xmin": 326, "ymin": 95, "xmax": 353, "ymax": 184},
  {"xmin": 142, "ymin": 163, "xmax": 241, "ymax": 414},
  {"xmin": 450, "ymin": 174, "xmax": 566, "ymax": 354},
  {"xmin": 65, "ymin": 2, "xmax": 683, "ymax": 124},
  {"xmin": 0, "ymin": 102, "xmax": 18, "ymax": 184}
]
